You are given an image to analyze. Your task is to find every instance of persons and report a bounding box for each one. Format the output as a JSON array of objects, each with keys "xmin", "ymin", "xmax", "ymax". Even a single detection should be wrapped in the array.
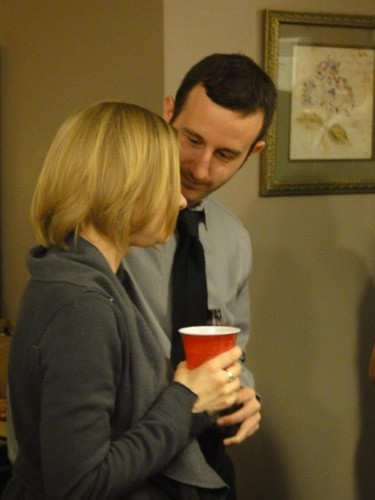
[
  {"xmin": 122, "ymin": 53, "xmax": 276, "ymax": 500},
  {"xmin": 9, "ymin": 102, "xmax": 243, "ymax": 500}
]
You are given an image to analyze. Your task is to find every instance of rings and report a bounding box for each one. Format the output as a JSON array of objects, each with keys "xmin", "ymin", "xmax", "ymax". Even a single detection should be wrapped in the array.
[{"xmin": 224, "ymin": 367, "xmax": 236, "ymax": 385}]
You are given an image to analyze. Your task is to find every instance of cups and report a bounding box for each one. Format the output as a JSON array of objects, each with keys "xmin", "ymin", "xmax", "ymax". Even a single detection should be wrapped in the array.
[{"xmin": 178, "ymin": 326, "xmax": 242, "ymax": 369}]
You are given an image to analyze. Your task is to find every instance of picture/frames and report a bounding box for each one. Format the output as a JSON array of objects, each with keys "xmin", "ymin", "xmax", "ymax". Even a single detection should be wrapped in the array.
[{"xmin": 258, "ymin": 8, "xmax": 375, "ymax": 198}]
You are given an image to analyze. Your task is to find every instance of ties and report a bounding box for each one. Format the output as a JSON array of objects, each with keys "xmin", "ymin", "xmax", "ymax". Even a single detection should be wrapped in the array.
[{"xmin": 169, "ymin": 208, "xmax": 237, "ymax": 495}]
